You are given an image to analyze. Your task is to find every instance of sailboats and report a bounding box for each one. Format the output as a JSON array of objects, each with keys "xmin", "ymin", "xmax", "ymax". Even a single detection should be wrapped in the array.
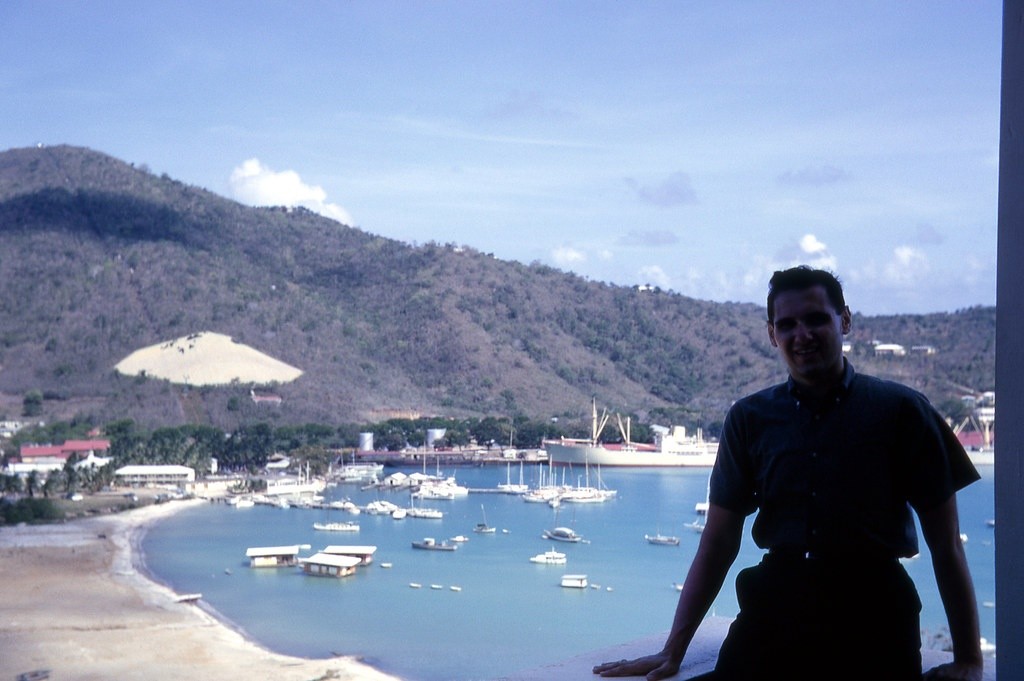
[
  {"xmin": 471, "ymin": 505, "xmax": 496, "ymax": 533},
  {"xmin": 497, "ymin": 454, "xmax": 617, "ymax": 508},
  {"xmin": 681, "ymin": 469, "xmax": 712, "ymax": 532},
  {"xmin": 287, "ymin": 447, "xmax": 386, "ymax": 493}
]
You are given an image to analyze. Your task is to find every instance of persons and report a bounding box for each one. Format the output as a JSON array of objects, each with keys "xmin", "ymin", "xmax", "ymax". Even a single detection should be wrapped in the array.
[{"xmin": 591, "ymin": 266, "xmax": 986, "ymax": 680}]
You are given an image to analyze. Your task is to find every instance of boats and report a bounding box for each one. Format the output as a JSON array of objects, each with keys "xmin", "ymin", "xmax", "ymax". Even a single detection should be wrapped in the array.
[
  {"xmin": 542, "ymin": 391, "xmax": 721, "ymax": 466},
  {"xmin": 313, "ymin": 521, "xmax": 359, "ymax": 532},
  {"xmin": 413, "ymin": 538, "xmax": 458, "ymax": 552},
  {"xmin": 644, "ymin": 535, "xmax": 680, "ymax": 546},
  {"xmin": 225, "ymin": 494, "xmax": 443, "ymax": 521},
  {"xmin": 449, "ymin": 536, "xmax": 469, "ymax": 541},
  {"xmin": 531, "ymin": 552, "xmax": 566, "ymax": 564},
  {"xmin": 411, "ymin": 476, "xmax": 468, "ymax": 500},
  {"xmin": 947, "ymin": 419, "xmax": 994, "ymax": 466},
  {"xmin": 545, "ymin": 525, "xmax": 583, "ymax": 543}
]
